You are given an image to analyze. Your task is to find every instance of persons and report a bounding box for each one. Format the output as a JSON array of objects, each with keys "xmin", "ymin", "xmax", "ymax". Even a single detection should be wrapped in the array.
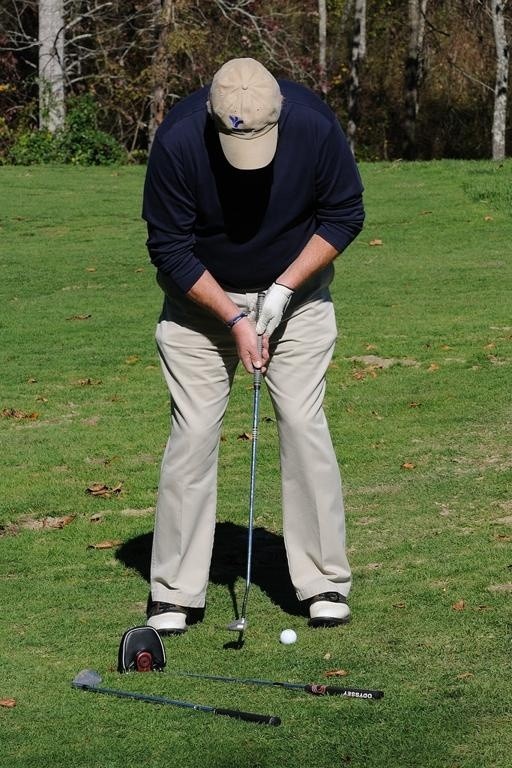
[{"xmin": 140, "ymin": 55, "xmax": 366, "ymax": 633}]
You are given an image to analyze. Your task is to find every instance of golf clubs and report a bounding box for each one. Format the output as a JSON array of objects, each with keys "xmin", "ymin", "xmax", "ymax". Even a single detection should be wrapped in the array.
[
  {"xmin": 71, "ymin": 669, "xmax": 281, "ymax": 730},
  {"xmin": 226, "ymin": 291, "xmax": 266, "ymax": 631}
]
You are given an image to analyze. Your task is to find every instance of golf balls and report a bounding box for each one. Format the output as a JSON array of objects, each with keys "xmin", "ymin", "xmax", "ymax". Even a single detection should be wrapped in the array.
[{"xmin": 280, "ymin": 628, "xmax": 297, "ymax": 647}]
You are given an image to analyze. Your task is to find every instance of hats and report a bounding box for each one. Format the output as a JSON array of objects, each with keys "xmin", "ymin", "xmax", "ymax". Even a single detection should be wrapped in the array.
[{"xmin": 205, "ymin": 56, "xmax": 285, "ymax": 170}]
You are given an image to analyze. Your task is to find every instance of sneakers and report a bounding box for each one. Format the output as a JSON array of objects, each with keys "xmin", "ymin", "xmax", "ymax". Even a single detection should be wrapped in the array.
[
  {"xmin": 308, "ymin": 592, "xmax": 351, "ymax": 626},
  {"xmin": 147, "ymin": 601, "xmax": 191, "ymax": 631}
]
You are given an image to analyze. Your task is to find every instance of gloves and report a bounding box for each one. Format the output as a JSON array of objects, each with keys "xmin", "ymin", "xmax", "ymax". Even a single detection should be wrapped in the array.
[{"xmin": 256, "ymin": 281, "xmax": 297, "ymax": 341}]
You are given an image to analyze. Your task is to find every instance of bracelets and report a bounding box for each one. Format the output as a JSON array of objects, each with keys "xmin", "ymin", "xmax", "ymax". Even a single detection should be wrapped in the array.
[{"xmin": 225, "ymin": 313, "xmax": 247, "ymax": 329}]
